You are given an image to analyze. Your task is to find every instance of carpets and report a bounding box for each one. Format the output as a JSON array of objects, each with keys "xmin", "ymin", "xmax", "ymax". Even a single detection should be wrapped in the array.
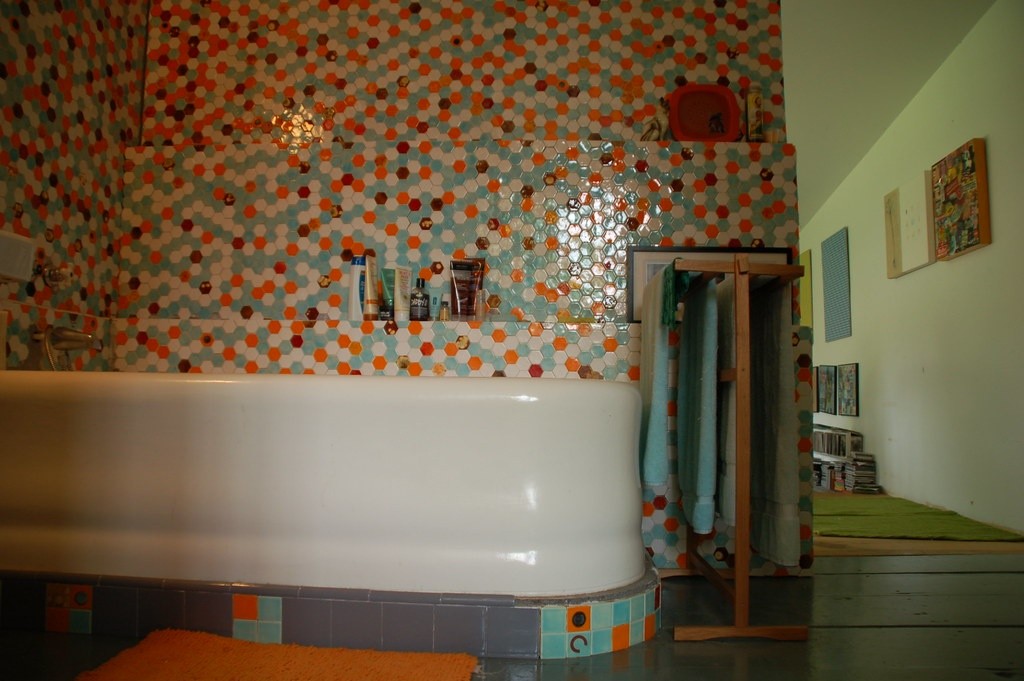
[
  {"xmin": 76, "ymin": 628, "xmax": 487, "ymax": 681},
  {"xmin": 812, "ymin": 497, "xmax": 1024, "ymax": 542}
]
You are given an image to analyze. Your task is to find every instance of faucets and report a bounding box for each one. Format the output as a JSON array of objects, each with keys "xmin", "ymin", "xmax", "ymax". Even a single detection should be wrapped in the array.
[{"xmin": 30, "ymin": 325, "xmax": 103, "ymax": 357}]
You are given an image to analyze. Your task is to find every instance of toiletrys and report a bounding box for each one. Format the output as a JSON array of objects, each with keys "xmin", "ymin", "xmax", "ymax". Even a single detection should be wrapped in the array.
[{"xmin": 350, "ymin": 254, "xmax": 487, "ymax": 322}]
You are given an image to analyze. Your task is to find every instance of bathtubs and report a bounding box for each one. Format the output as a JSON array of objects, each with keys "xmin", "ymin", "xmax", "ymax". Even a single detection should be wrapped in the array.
[{"xmin": 0, "ymin": 369, "xmax": 646, "ymax": 596}]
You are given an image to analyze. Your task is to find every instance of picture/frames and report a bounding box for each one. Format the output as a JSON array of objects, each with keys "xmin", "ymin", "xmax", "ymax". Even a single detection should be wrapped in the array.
[
  {"xmin": 837, "ymin": 363, "xmax": 859, "ymax": 418},
  {"xmin": 812, "ymin": 366, "xmax": 818, "ymax": 412},
  {"xmin": 817, "ymin": 365, "xmax": 837, "ymax": 415}
]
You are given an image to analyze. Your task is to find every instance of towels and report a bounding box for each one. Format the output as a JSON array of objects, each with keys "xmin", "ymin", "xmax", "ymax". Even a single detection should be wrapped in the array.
[
  {"xmin": 639, "ymin": 258, "xmax": 718, "ymax": 535},
  {"xmin": 719, "ymin": 271, "xmax": 801, "ymax": 569}
]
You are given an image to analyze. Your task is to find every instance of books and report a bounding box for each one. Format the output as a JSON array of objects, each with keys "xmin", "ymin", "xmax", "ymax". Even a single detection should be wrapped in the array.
[{"xmin": 812, "ymin": 424, "xmax": 882, "ymax": 496}]
